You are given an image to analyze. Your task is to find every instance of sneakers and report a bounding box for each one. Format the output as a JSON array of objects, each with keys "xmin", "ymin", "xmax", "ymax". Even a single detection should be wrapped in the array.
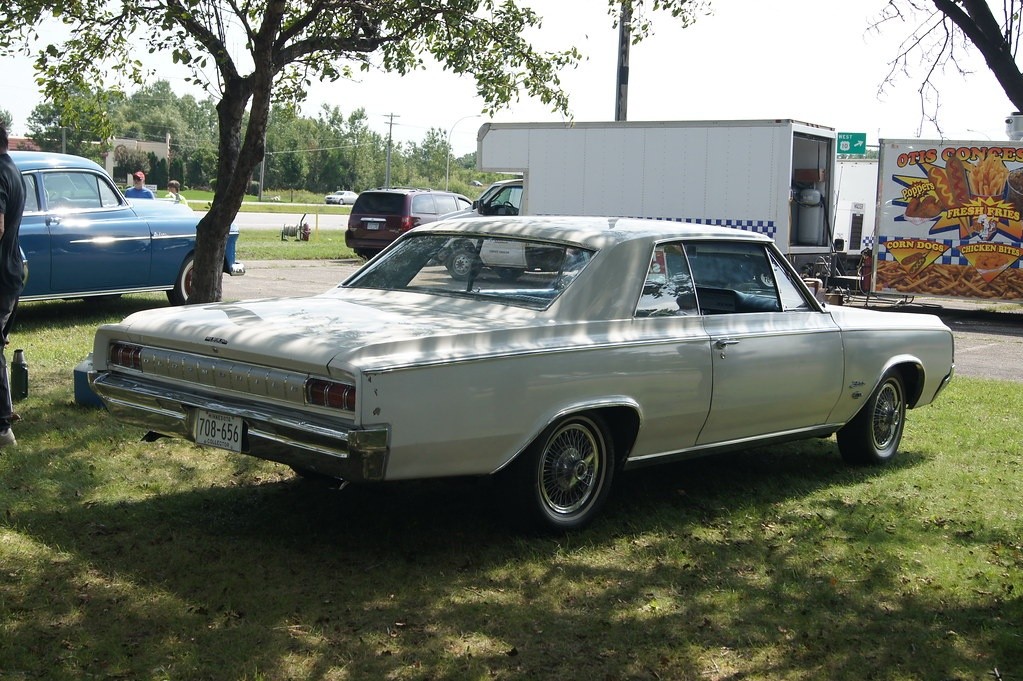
[{"xmin": 0, "ymin": 428, "xmax": 17, "ymax": 448}]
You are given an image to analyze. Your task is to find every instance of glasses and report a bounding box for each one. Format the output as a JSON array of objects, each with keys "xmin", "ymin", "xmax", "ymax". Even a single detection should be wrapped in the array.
[{"xmin": 133, "ymin": 178, "xmax": 144, "ymax": 181}]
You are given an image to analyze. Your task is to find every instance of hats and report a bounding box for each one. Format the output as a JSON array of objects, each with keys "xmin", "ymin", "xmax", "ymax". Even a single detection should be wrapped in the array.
[{"xmin": 133, "ymin": 171, "xmax": 145, "ymax": 178}]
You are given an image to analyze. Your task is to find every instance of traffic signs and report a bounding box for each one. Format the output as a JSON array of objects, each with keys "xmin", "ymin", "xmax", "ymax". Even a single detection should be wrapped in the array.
[{"xmin": 837, "ymin": 132, "xmax": 867, "ymax": 154}]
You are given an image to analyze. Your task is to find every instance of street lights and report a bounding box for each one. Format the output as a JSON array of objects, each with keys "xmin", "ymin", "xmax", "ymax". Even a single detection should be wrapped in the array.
[{"xmin": 445, "ymin": 113, "xmax": 481, "ymax": 192}]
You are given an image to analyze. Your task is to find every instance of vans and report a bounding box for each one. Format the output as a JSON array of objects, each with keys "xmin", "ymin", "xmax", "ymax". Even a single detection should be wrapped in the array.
[{"xmin": 343, "ymin": 185, "xmax": 475, "ymax": 261}]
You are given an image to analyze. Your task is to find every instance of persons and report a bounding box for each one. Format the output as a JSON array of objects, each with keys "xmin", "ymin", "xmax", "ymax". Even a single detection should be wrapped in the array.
[
  {"xmin": 163, "ymin": 178, "xmax": 189, "ymax": 207},
  {"xmin": 122, "ymin": 170, "xmax": 154, "ymax": 198},
  {"xmin": 0, "ymin": 123, "xmax": 31, "ymax": 451}
]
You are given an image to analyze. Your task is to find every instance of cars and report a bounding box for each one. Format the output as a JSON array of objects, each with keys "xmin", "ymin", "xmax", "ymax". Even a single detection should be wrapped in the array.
[
  {"xmin": 2, "ymin": 149, "xmax": 246, "ymax": 307},
  {"xmin": 325, "ymin": 191, "xmax": 359, "ymax": 205},
  {"xmin": 71, "ymin": 213, "xmax": 958, "ymax": 532}
]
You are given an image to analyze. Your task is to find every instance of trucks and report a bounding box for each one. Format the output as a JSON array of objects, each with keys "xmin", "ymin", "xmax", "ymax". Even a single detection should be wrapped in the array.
[{"xmin": 437, "ymin": 119, "xmax": 839, "ymax": 282}]
